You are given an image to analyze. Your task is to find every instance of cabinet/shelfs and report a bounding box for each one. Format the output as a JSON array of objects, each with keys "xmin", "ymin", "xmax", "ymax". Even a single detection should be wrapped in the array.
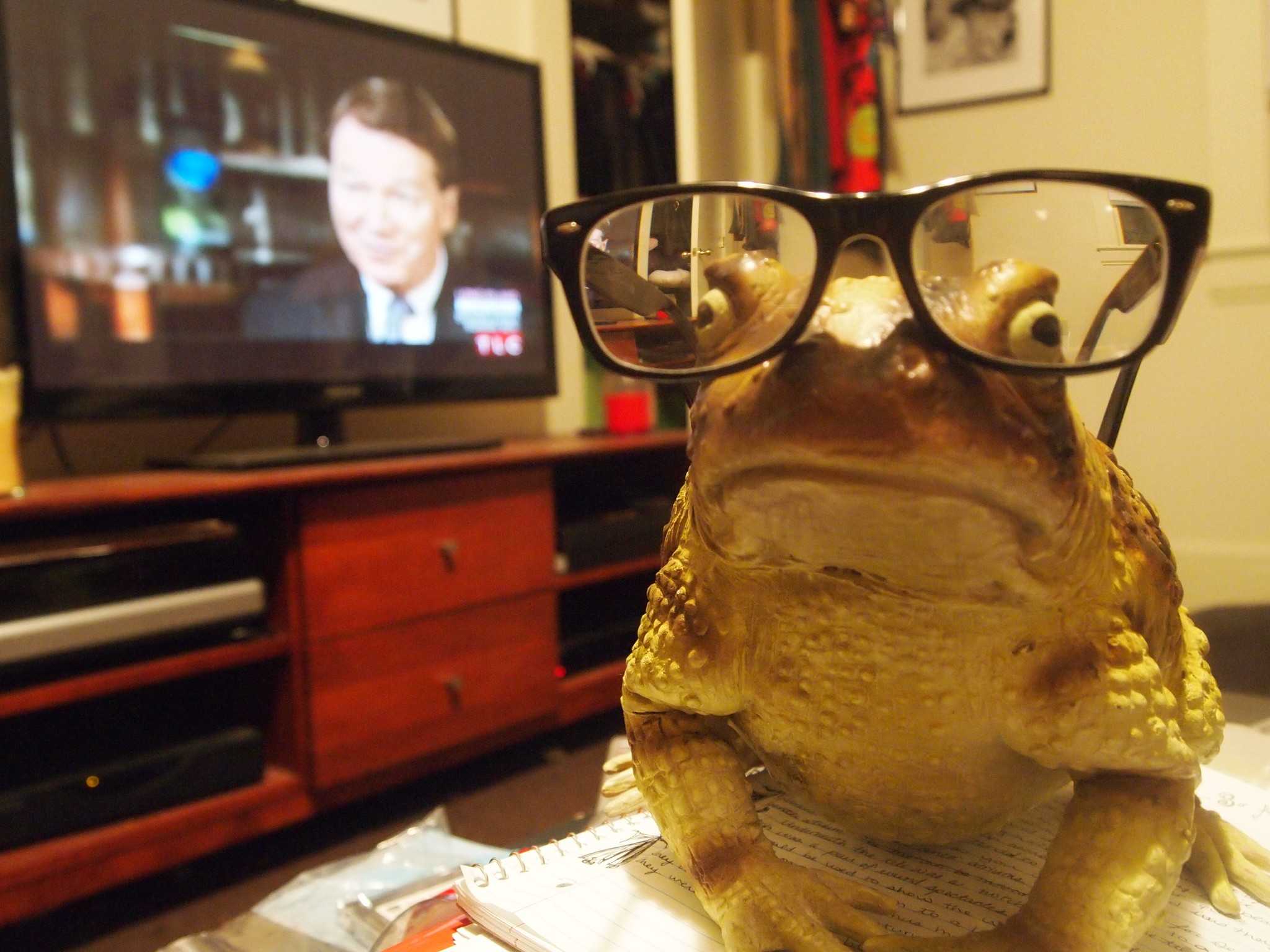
[{"xmin": 1, "ymin": 430, "xmax": 689, "ymax": 929}]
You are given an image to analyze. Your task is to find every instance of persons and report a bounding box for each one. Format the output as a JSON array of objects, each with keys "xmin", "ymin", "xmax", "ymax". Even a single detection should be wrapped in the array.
[{"xmin": 239, "ymin": 75, "xmax": 547, "ymax": 346}]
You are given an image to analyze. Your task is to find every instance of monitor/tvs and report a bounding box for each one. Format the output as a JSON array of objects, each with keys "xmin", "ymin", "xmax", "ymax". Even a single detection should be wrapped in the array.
[{"xmin": 0, "ymin": 0, "xmax": 561, "ymax": 473}]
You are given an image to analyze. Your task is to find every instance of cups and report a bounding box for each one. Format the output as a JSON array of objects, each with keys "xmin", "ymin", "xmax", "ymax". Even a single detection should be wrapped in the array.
[{"xmin": 602, "ymin": 367, "xmax": 656, "ymax": 435}]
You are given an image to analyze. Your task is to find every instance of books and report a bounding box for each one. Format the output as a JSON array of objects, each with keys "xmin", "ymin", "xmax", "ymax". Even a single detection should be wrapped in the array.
[{"xmin": 454, "ymin": 809, "xmax": 731, "ymax": 952}]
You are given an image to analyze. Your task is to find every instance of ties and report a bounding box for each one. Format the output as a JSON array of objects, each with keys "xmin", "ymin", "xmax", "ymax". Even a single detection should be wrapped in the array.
[{"xmin": 379, "ymin": 295, "xmax": 413, "ymax": 345}]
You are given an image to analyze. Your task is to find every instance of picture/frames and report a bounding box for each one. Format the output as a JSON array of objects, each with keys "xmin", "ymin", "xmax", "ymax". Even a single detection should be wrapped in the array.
[{"xmin": 893, "ymin": 0, "xmax": 1052, "ymax": 118}]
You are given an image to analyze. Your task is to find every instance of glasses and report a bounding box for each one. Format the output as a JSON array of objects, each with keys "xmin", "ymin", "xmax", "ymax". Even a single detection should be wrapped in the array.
[{"xmin": 539, "ymin": 166, "xmax": 1212, "ymax": 454}]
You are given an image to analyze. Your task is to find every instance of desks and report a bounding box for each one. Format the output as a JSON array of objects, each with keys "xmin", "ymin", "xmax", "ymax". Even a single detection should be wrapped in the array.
[{"xmin": 88, "ymin": 734, "xmax": 617, "ymax": 952}]
